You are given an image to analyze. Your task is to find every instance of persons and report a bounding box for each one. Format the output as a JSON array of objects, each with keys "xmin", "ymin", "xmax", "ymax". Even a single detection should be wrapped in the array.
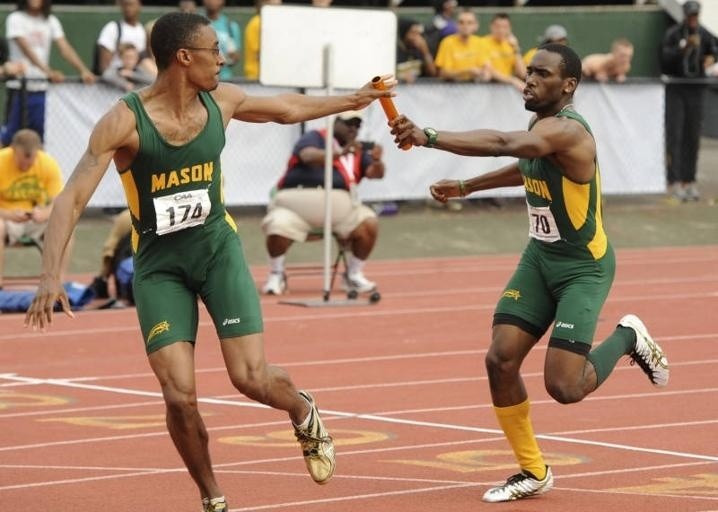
[
  {"xmin": 1, "ymin": 2, "xmax": 281, "ymax": 212},
  {"xmin": 261, "ymin": 115, "xmax": 379, "ymax": 293},
  {"xmin": 387, "ymin": 45, "xmax": 670, "ymax": 505},
  {"xmin": 661, "ymin": 1, "xmax": 718, "ymax": 200},
  {"xmin": 1, "ymin": 129, "xmax": 64, "ymax": 247},
  {"xmin": 311, "ymin": 0, "xmax": 633, "ymax": 213},
  {"xmin": 21, "ymin": 15, "xmax": 399, "ymax": 512}
]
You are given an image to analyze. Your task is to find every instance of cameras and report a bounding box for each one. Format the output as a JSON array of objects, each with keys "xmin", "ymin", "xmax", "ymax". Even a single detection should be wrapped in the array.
[{"xmin": 360, "ymin": 141, "xmax": 374, "ymax": 150}]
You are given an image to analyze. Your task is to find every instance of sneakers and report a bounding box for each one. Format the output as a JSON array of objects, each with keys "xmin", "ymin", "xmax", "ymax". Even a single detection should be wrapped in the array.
[
  {"xmin": 260, "ymin": 272, "xmax": 285, "ymax": 294},
  {"xmin": 616, "ymin": 315, "xmax": 668, "ymax": 388},
  {"xmin": 203, "ymin": 495, "xmax": 230, "ymax": 512},
  {"xmin": 668, "ymin": 181, "xmax": 700, "ymax": 206},
  {"xmin": 482, "ymin": 466, "xmax": 554, "ymax": 502},
  {"xmin": 292, "ymin": 392, "xmax": 335, "ymax": 484},
  {"xmin": 342, "ymin": 272, "xmax": 375, "ymax": 294}
]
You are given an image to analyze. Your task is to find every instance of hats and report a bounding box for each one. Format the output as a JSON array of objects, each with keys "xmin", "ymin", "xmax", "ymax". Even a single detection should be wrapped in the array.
[
  {"xmin": 11, "ymin": 130, "xmax": 41, "ymax": 153},
  {"xmin": 341, "ymin": 109, "xmax": 364, "ymax": 122}
]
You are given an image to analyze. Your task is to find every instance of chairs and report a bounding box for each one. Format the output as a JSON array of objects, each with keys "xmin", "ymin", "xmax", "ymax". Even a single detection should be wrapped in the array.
[{"xmin": 267, "ymin": 184, "xmax": 350, "ymax": 291}]
